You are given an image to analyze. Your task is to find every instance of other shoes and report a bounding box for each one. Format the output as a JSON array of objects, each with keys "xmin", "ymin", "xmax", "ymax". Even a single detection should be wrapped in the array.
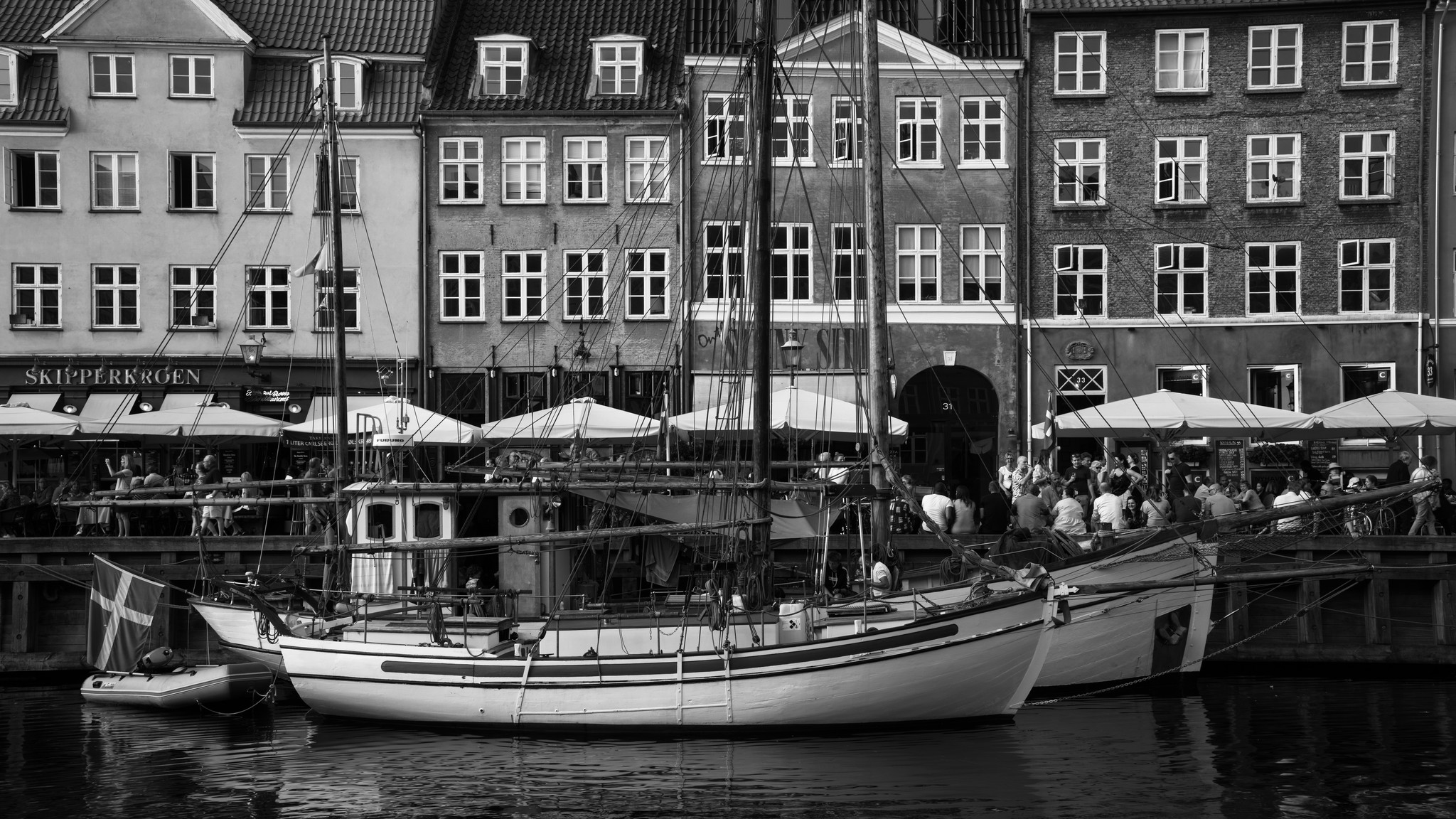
[
  {"xmin": 2, "ymin": 534, "xmax": 16, "ymax": 538},
  {"xmin": 234, "ymin": 529, "xmax": 246, "ymax": 536}
]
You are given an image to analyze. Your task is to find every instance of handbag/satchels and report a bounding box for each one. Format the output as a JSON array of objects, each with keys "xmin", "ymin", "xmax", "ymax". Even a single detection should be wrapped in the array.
[
  {"xmin": 1001, "ymin": 490, "xmax": 1013, "ymax": 505},
  {"xmin": 1434, "ymin": 489, "xmax": 1447, "ymax": 508}
]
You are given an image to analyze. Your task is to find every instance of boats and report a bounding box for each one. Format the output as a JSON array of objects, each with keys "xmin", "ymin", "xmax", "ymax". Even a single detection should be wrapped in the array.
[{"xmin": 78, "ymin": 647, "xmax": 273, "ymax": 712}]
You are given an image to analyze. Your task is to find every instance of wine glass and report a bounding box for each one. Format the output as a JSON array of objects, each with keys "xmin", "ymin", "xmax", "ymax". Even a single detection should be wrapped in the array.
[
  {"xmin": 1111, "ymin": 469, "xmax": 1116, "ymax": 480},
  {"xmin": 1026, "ymin": 464, "xmax": 1033, "ymax": 472},
  {"xmin": 1232, "ymin": 494, "xmax": 1236, "ymax": 502},
  {"xmin": 1072, "ymin": 472, "xmax": 1076, "ymax": 484}
]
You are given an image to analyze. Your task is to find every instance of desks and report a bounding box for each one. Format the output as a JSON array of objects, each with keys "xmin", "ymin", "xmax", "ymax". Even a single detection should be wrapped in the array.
[{"xmin": 76, "ymin": 507, "xmax": 113, "ymax": 536}]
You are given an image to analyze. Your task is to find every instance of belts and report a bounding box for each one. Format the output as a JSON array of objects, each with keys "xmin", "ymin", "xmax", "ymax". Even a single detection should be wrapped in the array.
[{"xmin": 204, "ymin": 490, "xmax": 220, "ymax": 496}]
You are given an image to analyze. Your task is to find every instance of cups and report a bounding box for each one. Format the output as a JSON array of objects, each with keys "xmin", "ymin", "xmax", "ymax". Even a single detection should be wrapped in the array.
[{"xmin": 1092, "ymin": 479, "xmax": 1096, "ymax": 487}]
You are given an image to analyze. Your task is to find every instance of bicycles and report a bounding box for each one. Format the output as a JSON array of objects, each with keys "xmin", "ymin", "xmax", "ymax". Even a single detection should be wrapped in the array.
[
  {"xmin": 1337, "ymin": 489, "xmax": 1372, "ymax": 537},
  {"xmin": 1363, "ymin": 487, "xmax": 1396, "ymax": 536}
]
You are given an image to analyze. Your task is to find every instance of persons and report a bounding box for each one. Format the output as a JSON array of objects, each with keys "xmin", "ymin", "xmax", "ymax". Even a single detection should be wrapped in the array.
[
  {"xmin": 0, "ymin": 477, "xmax": 96, "ymax": 538},
  {"xmin": 818, "ymin": 551, "xmax": 852, "ymax": 605},
  {"xmin": 709, "ymin": 454, "xmax": 727, "ymax": 497},
  {"xmin": 302, "ymin": 457, "xmax": 339, "ymax": 536},
  {"xmin": 568, "ymin": 443, "xmax": 627, "ymax": 474},
  {"xmin": 484, "ymin": 450, "xmax": 554, "ymax": 483},
  {"xmin": 1171, "ymin": 461, "xmax": 1382, "ymax": 540},
  {"xmin": 1383, "ymin": 451, "xmax": 1456, "ymax": 535},
  {"xmin": 859, "ymin": 553, "xmax": 893, "ymax": 600},
  {"xmin": 889, "ymin": 474, "xmax": 1009, "ymax": 536},
  {"xmin": 229, "ymin": 471, "xmax": 258, "ymax": 536},
  {"xmin": 466, "ymin": 562, "xmax": 488, "ymax": 616},
  {"xmin": 801, "ymin": 450, "xmax": 849, "ymax": 487},
  {"xmin": 184, "ymin": 455, "xmax": 225, "ymax": 537},
  {"xmin": 997, "ymin": 449, "xmax": 1194, "ymax": 536},
  {"xmin": 104, "ymin": 454, "xmax": 184, "ymax": 538}
]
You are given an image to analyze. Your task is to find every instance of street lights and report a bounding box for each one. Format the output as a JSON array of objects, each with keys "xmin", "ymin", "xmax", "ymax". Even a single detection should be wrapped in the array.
[{"xmin": 779, "ymin": 324, "xmax": 803, "ymax": 385}]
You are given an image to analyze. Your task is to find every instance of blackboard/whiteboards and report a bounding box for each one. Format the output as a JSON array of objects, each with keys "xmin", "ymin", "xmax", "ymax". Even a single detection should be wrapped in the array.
[
  {"xmin": 1215, "ymin": 439, "xmax": 1245, "ymax": 489},
  {"xmin": 1307, "ymin": 439, "xmax": 1338, "ymax": 478}
]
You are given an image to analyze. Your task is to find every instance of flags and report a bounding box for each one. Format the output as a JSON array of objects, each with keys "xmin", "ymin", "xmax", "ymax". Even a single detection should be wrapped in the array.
[
  {"xmin": 85, "ymin": 554, "xmax": 163, "ymax": 673},
  {"xmin": 1043, "ymin": 397, "xmax": 1058, "ymax": 458},
  {"xmin": 290, "ymin": 239, "xmax": 328, "ymax": 278}
]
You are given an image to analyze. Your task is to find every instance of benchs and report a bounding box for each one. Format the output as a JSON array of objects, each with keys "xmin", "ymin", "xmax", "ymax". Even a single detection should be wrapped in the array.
[{"xmin": 234, "ymin": 496, "xmax": 287, "ymax": 535}]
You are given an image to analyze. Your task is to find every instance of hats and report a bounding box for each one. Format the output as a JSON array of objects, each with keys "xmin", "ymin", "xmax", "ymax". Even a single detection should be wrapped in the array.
[
  {"xmin": 1347, "ymin": 477, "xmax": 1360, "ymax": 488},
  {"xmin": 1327, "ymin": 462, "xmax": 1343, "ymax": 473}
]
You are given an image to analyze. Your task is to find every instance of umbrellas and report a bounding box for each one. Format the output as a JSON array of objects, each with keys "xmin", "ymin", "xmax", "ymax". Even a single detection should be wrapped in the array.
[
  {"xmin": 280, "ymin": 398, "xmax": 490, "ymax": 458},
  {"xmin": 80, "ymin": 402, "xmax": 299, "ymax": 451},
  {"xmin": 480, "ymin": 398, "xmax": 666, "ymax": 448},
  {"xmin": 1031, "ymin": 387, "xmax": 1317, "ymax": 498},
  {"xmin": 0, "ymin": 402, "xmax": 99, "ymax": 487},
  {"xmin": 668, "ymin": 384, "xmax": 909, "ymax": 482},
  {"xmin": 1311, "ymin": 388, "xmax": 1456, "ymax": 464}
]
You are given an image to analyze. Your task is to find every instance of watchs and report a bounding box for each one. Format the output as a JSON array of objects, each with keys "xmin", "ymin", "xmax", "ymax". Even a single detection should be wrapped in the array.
[{"xmin": 521, "ymin": 456, "xmax": 525, "ymax": 461}]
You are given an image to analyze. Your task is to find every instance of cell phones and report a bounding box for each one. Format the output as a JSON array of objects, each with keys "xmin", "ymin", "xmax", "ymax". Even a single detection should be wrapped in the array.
[
  {"xmin": 1129, "ymin": 515, "xmax": 1133, "ymax": 519},
  {"xmin": 1331, "ymin": 474, "xmax": 1340, "ymax": 480}
]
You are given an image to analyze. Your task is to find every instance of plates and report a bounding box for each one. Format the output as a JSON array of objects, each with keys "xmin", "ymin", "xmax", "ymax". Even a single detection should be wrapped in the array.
[
  {"xmin": 854, "ymin": 578, "xmax": 871, "ymax": 581},
  {"xmin": 796, "ymin": 600, "xmax": 809, "ymax": 602}
]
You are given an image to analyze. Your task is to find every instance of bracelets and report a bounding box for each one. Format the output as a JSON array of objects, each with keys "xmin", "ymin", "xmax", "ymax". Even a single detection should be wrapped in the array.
[{"xmin": 239, "ymin": 496, "xmax": 241, "ymax": 498}]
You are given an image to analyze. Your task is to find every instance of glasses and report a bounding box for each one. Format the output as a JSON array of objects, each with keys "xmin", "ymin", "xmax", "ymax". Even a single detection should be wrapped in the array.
[
  {"xmin": 39, "ymin": 481, "xmax": 46, "ymax": 484},
  {"xmin": 510, "ymin": 456, "xmax": 519, "ymax": 458},
  {"xmin": 194, "ymin": 467, "xmax": 199, "ymax": 469},
  {"xmin": 3, "ymin": 482, "xmax": 10, "ymax": 486},
  {"xmin": 1034, "ymin": 491, "xmax": 1041, "ymax": 494},
  {"xmin": 132, "ymin": 484, "xmax": 140, "ymax": 487},
  {"xmin": 486, "ymin": 463, "xmax": 491, "ymax": 465},
  {"xmin": 203, "ymin": 461, "xmax": 209, "ymax": 463},
  {"xmin": 240, "ymin": 479, "xmax": 241, "ymax": 481},
  {"xmin": 1208, "ymin": 482, "xmax": 1215, "ymax": 484},
  {"xmin": 1104, "ymin": 459, "xmax": 1106, "ymax": 461},
  {"xmin": 1096, "ymin": 467, "xmax": 1102, "ymax": 469},
  {"xmin": 1167, "ymin": 455, "xmax": 1178, "ymax": 461},
  {"xmin": 1319, "ymin": 489, "xmax": 1327, "ymax": 492},
  {"xmin": 1006, "ymin": 458, "xmax": 1013, "ymax": 460},
  {"xmin": 1072, "ymin": 454, "xmax": 1080, "ymax": 457},
  {"xmin": 1208, "ymin": 488, "xmax": 1214, "ymax": 493},
  {"xmin": 1219, "ymin": 473, "xmax": 1227, "ymax": 479}
]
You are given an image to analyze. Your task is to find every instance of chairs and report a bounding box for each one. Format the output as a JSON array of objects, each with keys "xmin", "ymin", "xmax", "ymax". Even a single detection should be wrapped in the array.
[
  {"xmin": 128, "ymin": 508, "xmax": 193, "ymax": 537},
  {"xmin": 1263, "ymin": 493, "xmax": 1275, "ymax": 510},
  {"xmin": 0, "ymin": 499, "xmax": 79, "ymax": 537}
]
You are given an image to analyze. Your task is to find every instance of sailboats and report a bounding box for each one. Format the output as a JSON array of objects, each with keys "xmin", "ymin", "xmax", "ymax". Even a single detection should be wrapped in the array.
[{"xmin": 51, "ymin": 2, "xmax": 1452, "ymax": 734}]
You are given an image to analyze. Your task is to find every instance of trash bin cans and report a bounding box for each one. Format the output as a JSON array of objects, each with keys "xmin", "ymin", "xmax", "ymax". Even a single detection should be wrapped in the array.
[{"xmin": 779, "ymin": 604, "xmax": 812, "ymax": 644}]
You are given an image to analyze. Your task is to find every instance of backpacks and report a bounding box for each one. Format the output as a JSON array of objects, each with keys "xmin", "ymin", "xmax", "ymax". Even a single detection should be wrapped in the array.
[
  {"xmin": 808, "ymin": 468, "xmax": 823, "ymax": 481},
  {"xmin": 20, "ymin": 495, "xmax": 31, "ymax": 505}
]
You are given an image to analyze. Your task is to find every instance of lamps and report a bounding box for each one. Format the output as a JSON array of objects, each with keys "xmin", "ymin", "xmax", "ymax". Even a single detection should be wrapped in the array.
[
  {"xmin": 288, "ymin": 404, "xmax": 302, "ymax": 413},
  {"xmin": 133, "ymin": 358, "xmax": 146, "ymax": 373},
  {"xmin": 219, "ymin": 402, "xmax": 230, "ymax": 409},
  {"xmin": 63, "ymin": 404, "xmax": 77, "ymax": 414},
  {"xmin": 491, "ymin": 370, "xmax": 495, "ymax": 378},
  {"xmin": 614, "ymin": 368, "xmax": 619, "ymax": 376},
  {"xmin": 552, "ymin": 369, "xmax": 557, "ymax": 376},
  {"xmin": 65, "ymin": 357, "xmax": 80, "ymax": 375},
  {"xmin": 164, "ymin": 357, "xmax": 179, "ymax": 375},
  {"xmin": 542, "ymin": 494, "xmax": 562, "ymax": 520},
  {"xmin": 140, "ymin": 402, "xmax": 153, "ymax": 411},
  {"xmin": 429, "ymin": 370, "xmax": 434, "ymax": 378},
  {"xmin": 31, "ymin": 357, "xmax": 45, "ymax": 376},
  {"xmin": 236, "ymin": 330, "xmax": 273, "ymax": 385},
  {"xmin": 99, "ymin": 358, "xmax": 114, "ymax": 376},
  {"xmin": 674, "ymin": 369, "xmax": 679, "ymax": 376}
]
